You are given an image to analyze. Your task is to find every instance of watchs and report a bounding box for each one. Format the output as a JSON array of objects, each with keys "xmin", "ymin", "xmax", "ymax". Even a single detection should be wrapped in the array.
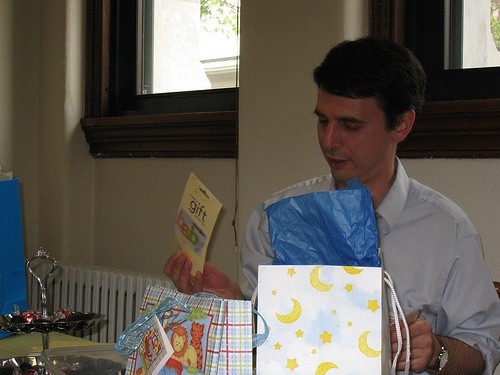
[{"xmin": 422, "ymin": 332, "xmax": 450, "ymax": 371}]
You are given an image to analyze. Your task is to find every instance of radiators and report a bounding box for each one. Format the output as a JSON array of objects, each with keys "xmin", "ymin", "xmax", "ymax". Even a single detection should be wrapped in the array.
[{"xmin": 29, "ymin": 256, "xmax": 178, "ymax": 342}]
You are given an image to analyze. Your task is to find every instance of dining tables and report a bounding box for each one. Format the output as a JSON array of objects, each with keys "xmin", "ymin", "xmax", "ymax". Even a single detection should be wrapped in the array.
[{"xmin": 1, "ymin": 330, "xmax": 131, "ymax": 375}]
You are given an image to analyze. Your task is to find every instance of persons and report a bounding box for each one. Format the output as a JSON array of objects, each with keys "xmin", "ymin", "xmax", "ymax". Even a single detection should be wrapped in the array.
[{"xmin": 162, "ymin": 38, "xmax": 500, "ymax": 374}]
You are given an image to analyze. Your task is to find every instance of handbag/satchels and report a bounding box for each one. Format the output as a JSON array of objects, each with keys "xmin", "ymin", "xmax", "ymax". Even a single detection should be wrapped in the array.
[
  {"xmin": 115, "ymin": 284, "xmax": 255, "ymax": 375},
  {"xmin": 258, "ymin": 247, "xmax": 411, "ymax": 375}
]
